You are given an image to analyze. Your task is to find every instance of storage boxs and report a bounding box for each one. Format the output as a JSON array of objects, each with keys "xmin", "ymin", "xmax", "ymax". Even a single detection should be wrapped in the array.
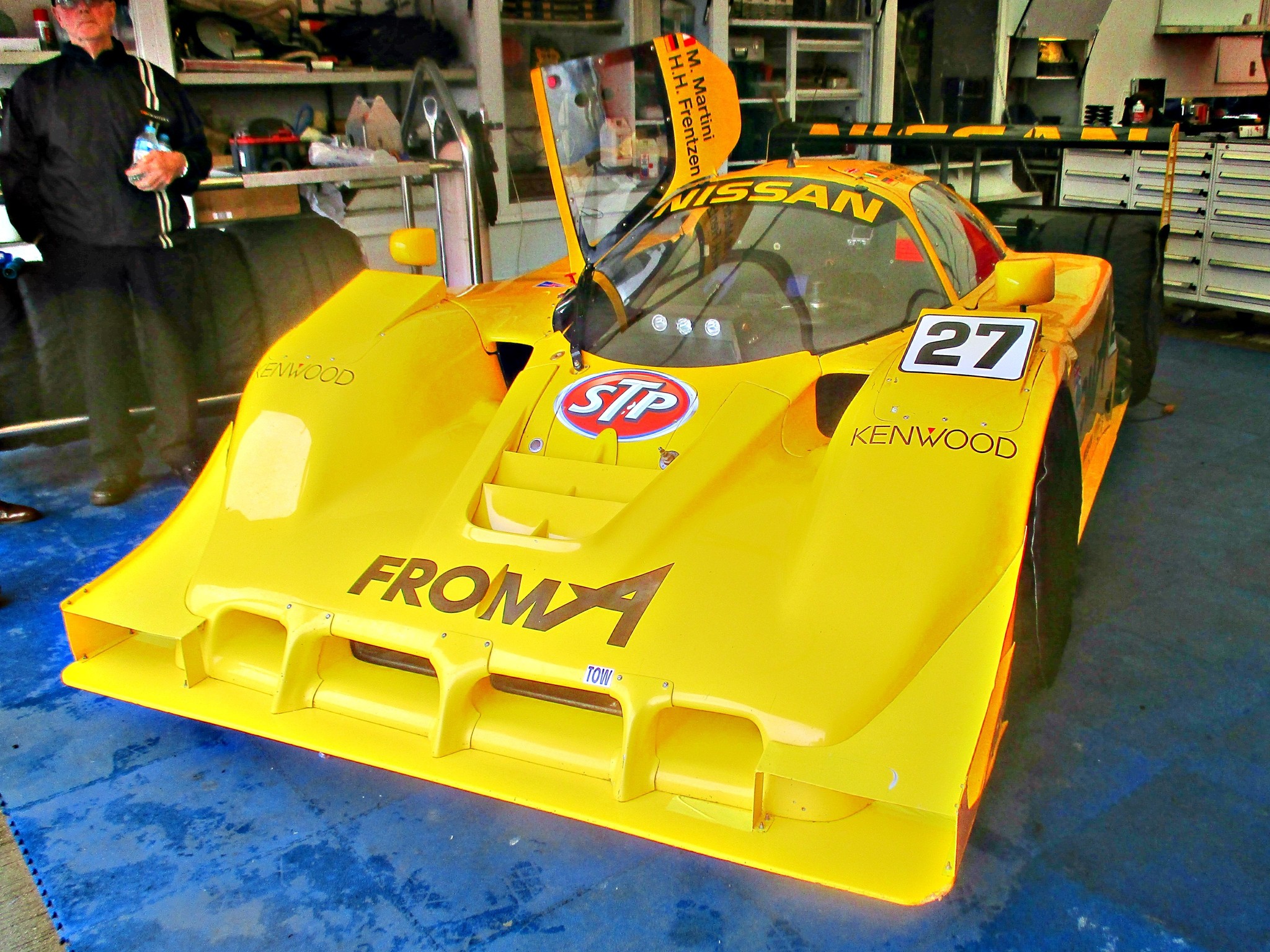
[{"xmin": 197, "ymin": 184, "xmax": 300, "ymax": 225}]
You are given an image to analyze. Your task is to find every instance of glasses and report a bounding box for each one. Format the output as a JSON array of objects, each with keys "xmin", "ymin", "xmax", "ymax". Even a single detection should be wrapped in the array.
[{"xmin": 56, "ymin": 0, "xmax": 108, "ymax": 10}]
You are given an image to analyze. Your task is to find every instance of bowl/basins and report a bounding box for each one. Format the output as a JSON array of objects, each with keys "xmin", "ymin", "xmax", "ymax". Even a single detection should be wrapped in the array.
[
  {"xmin": 509, "ymin": 154, "xmax": 535, "ymax": 173},
  {"xmin": 827, "ymin": 78, "xmax": 848, "ymax": 89},
  {"xmin": 731, "ymin": 47, "xmax": 748, "ymax": 60},
  {"xmin": 1239, "ymin": 114, "xmax": 1258, "ymax": 119}
]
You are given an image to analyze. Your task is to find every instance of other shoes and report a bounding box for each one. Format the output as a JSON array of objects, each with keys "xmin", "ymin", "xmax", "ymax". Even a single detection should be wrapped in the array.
[
  {"xmin": 172, "ymin": 459, "xmax": 202, "ymax": 486},
  {"xmin": 91, "ymin": 471, "xmax": 138, "ymax": 504}
]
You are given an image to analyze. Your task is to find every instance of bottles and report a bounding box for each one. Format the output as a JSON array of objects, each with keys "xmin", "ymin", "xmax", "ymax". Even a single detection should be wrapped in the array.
[
  {"xmin": 32, "ymin": 9, "xmax": 58, "ymax": 52},
  {"xmin": 1133, "ymin": 100, "xmax": 1144, "ymax": 123},
  {"xmin": 309, "ymin": 143, "xmax": 398, "ymax": 167},
  {"xmin": 155, "ymin": 134, "xmax": 173, "ymax": 152},
  {"xmin": 534, "ymin": 117, "xmax": 549, "ymax": 167},
  {"xmin": 128, "ymin": 125, "xmax": 159, "ymax": 186},
  {"xmin": 842, "ymin": 106, "xmax": 854, "ymax": 154}
]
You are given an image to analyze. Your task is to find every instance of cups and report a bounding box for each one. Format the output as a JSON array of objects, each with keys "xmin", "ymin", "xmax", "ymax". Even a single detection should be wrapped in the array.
[
  {"xmin": 231, "ymin": 47, "xmax": 261, "ymax": 61},
  {"xmin": 3, "ymin": 257, "xmax": 25, "ymax": 279}
]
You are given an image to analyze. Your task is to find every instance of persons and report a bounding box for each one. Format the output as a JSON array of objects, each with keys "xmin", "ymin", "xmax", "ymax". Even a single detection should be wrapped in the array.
[{"xmin": 0, "ymin": 0, "xmax": 215, "ymax": 506}]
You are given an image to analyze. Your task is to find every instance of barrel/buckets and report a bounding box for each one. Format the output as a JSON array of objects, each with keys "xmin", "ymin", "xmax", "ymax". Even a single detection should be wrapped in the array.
[
  {"xmin": 599, "ymin": 118, "xmax": 632, "ymax": 167},
  {"xmin": 636, "ymin": 139, "xmax": 659, "ymax": 179}
]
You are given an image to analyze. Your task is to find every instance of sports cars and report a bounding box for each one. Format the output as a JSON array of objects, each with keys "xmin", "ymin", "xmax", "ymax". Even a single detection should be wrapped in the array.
[{"xmin": 57, "ymin": 34, "xmax": 1180, "ymax": 910}]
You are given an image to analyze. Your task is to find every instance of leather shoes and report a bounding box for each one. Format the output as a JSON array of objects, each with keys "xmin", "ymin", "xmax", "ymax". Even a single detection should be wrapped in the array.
[{"xmin": 0, "ymin": 500, "xmax": 40, "ymax": 524}]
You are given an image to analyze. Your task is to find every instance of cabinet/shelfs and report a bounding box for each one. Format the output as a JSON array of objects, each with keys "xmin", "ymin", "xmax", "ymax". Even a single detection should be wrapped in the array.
[{"xmin": 0, "ymin": 0, "xmax": 876, "ymax": 279}]
